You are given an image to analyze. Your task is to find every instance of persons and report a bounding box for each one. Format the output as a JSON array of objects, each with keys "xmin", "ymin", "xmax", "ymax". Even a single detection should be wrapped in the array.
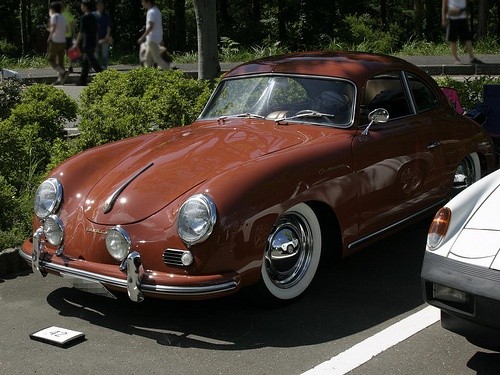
[
  {"xmin": 441, "ymin": 0, "xmax": 486, "ymax": 64},
  {"xmin": 137, "ymin": 0, "xmax": 173, "ymax": 72},
  {"xmin": 71, "ymin": 0, "xmax": 104, "ymax": 86},
  {"xmin": 90, "ymin": 0, "xmax": 111, "ymax": 69},
  {"xmin": 46, "ymin": 1, "xmax": 69, "ymax": 85},
  {"xmin": 60, "ymin": 2, "xmax": 75, "ymax": 73}
]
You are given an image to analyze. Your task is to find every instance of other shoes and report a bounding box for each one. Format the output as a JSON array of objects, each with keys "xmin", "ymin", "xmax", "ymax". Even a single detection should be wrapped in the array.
[{"xmin": 68, "ymin": 67, "xmax": 73, "ymax": 72}]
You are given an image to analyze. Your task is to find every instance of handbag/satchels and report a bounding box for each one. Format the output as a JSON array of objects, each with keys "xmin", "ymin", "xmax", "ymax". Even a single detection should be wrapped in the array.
[
  {"xmin": 448, "ymin": 7, "xmax": 467, "ymax": 16},
  {"xmin": 68, "ymin": 47, "xmax": 81, "ymax": 62}
]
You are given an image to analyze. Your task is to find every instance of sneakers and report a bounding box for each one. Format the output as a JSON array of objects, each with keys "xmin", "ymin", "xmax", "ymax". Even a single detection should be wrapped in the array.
[
  {"xmin": 51, "ymin": 71, "xmax": 69, "ymax": 85},
  {"xmin": 75, "ymin": 80, "xmax": 88, "ymax": 86},
  {"xmin": 470, "ymin": 59, "xmax": 483, "ymax": 64}
]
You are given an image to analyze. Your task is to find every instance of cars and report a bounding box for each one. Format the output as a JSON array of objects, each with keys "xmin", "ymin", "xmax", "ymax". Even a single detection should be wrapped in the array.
[
  {"xmin": 18, "ymin": 50, "xmax": 498, "ymax": 315},
  {"xmin": 419, "ymin": 168, "xmax": 500, "ymax": 352}
]
